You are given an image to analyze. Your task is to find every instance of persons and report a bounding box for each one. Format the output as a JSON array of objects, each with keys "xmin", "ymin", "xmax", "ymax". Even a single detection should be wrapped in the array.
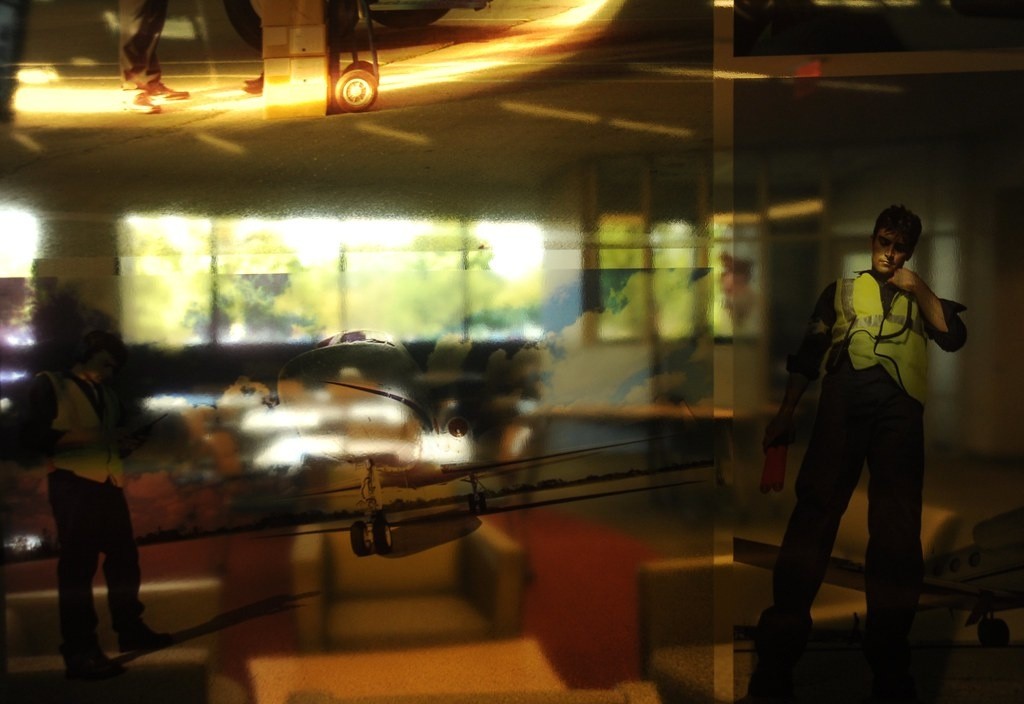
[
  {"xmin": 1, "ymin": 0, "xmax": 262, "ymax": 160},
  {"xmin": 27, "ymin": 331, "xmax": 172, "ymax": 681},
  {"xmin": 736, "ymin": 204, "xmax": 966, "ymax": 704}
]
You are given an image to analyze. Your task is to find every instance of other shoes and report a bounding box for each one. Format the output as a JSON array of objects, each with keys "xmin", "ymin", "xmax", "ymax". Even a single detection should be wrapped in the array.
[
  {"xmin": 123, "ymin": 88, "xmax": 161, "ymax": 113},
  {"xmin": 62, "ymin": 643, "xmax": 123, "ymax": 677},
  {"xmin": 150, "ymin": 82, "xmax": 188, "ymax": 99},
  {"xmin": 118, "ymin": 622, "xmax": 177, "ymax": 655}
]
